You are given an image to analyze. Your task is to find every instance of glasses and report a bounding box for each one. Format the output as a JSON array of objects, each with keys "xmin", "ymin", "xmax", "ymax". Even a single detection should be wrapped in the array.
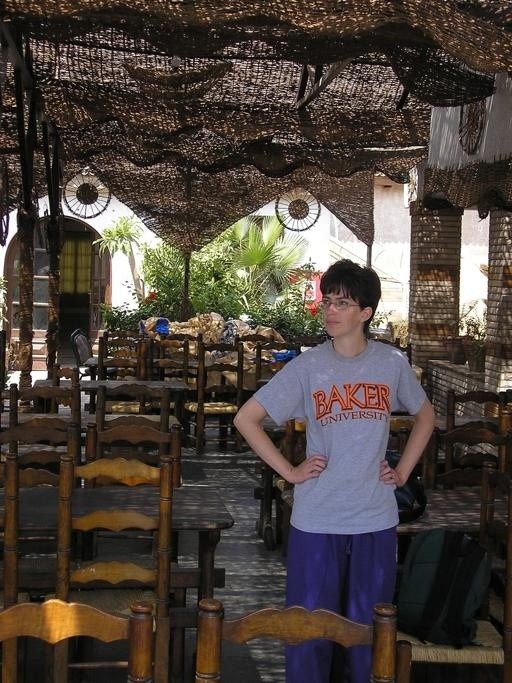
[{"xmin": 318, "ymin": 299, "xmax": 368, "ymax": 310}]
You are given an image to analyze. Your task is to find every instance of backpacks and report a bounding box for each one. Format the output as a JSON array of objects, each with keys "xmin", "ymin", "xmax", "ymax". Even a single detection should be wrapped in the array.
[
  {"xmin": 398, "ymin": 527, "xmax": 491, "ymax": 649},
  {"xmin": 384, "ymin": 452, "xmax": 427, "ymax": 523}
]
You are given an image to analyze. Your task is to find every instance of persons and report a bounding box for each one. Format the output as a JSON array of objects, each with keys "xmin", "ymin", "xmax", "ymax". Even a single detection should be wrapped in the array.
[{"xmin": 232, "ymin": 259, "xmax": 436, "ymax": 683}]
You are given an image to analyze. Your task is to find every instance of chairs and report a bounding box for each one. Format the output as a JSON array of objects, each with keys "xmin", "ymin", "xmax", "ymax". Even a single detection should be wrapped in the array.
[{"xmin": 0, "ymin": 331, "xmax": 511, "ymax": 683}]
